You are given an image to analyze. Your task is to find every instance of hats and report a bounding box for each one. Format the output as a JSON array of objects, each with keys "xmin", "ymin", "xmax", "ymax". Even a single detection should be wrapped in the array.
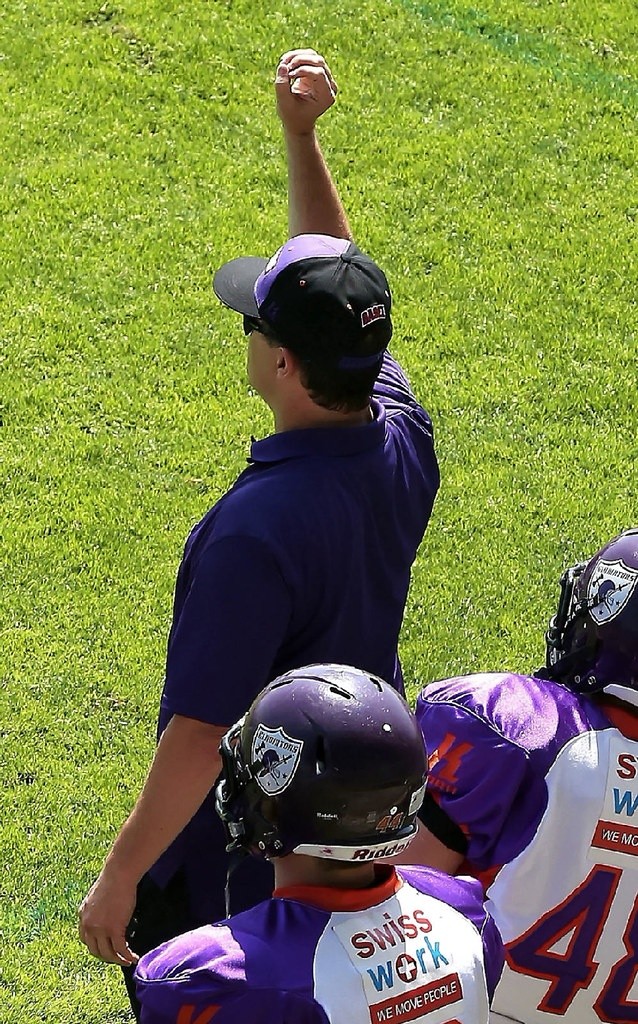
[{"xmin": 213, "ymin": 233, "xmax": 393, "ymax": 368}]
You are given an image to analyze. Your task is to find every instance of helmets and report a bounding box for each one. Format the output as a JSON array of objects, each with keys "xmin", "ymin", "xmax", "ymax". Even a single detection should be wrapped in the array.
[
  {"xmin": 215, "ymin": 663, "xmax": 430, "ymax": 857},
  {"xmin": 545, "ymin": 527, "xmax": 638, "ymax": 695}
]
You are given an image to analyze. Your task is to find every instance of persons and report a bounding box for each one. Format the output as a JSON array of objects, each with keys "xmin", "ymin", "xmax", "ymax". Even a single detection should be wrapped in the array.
[
  {"xmin": 373, "ymin": 530, "xmax": 638, "ymax": 1024},
  {"xmin": 77, "ymin": 47, "xmax": 441, "ymax": 997},
  {"xmin": 132, "ymin": 663, "xmax": 505, "ymax": 1024}
]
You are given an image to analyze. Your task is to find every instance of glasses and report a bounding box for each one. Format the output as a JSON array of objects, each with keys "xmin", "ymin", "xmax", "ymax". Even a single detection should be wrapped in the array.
[{"xmin": 243, "ymin": 315, "xmax": 294, "ymax": 354}]
